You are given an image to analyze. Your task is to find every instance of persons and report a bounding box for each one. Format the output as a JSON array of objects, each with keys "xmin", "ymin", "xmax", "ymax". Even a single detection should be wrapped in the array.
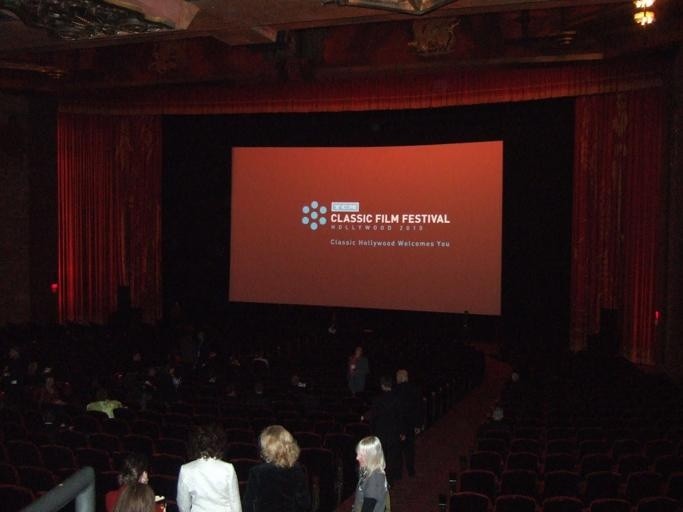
[
  {"xmin": 0, "ymin": 345, "xmax": 26, "ymax": 368},
  {"xmin": 103, "ymin": 451, "xmax": 169, "ymax": 512},
  {"xmin": 347, "ymin": 346, "xmax": 410, "ymax": 399},
  {"xmin": 85, "ymin": 387, "xmax": 128, "ymax": 420},
  {"xmin": 112, "ymin": 343, "xmax": 310, "ymax": 410},
  {"xmin": 240, "ymin": 422, "xmax": 312, "ymax": 512},
  {"xmin": 350, "ymin": 435, "xmax": 392, "ymax": 512},
  {"xmin": 37, "ymin": 370, "xmax": 68, "ymax": 405},
  {"xmin": 174, "ymin": 417, "xmax": 243, "ymax": 512}
]
